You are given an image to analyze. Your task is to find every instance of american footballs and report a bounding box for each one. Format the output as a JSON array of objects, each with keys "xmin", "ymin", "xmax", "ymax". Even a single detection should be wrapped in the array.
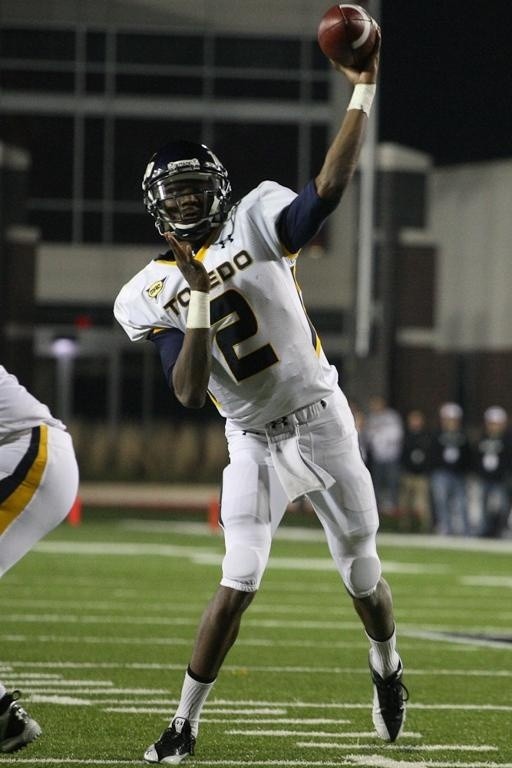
[{"xmin": 319, "ymin": 2, "xmax": 379, "ymax": 67}]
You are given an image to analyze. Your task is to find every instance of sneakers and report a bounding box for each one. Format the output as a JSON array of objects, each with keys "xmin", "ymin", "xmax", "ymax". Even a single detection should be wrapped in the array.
[
  {"xmin": 143, "ymin": 716, "xmax": 195, "ymax": 767},
  {"xmin": 0, "ymin": 690, "xmax": 44, "ymax": 754},
  {"xmin": 368, "ymin": 646, "xmax": 406, "ymax": 743}
]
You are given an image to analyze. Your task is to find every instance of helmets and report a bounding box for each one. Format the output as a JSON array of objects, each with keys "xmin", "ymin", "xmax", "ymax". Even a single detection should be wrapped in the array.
[{"xmin": 141, "ymin": 141, "xmax": 230, "ymax": 242}]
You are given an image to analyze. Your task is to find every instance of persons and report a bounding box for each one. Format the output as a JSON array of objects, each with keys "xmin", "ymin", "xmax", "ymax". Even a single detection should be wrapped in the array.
[
  {"xmin": 1, "ymin": 361, "xmax": 81, "ymax": 753},
  {"xmin": 351, "ymin": 392, "xmax": 510, "ymax": 538},
  {"xmin": 112, "ymin": 14, "xmax": 411, "ymax": 767}
]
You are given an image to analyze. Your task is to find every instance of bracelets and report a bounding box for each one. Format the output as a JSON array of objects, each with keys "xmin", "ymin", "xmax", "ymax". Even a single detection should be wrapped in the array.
[
  {"xmin": 187, "ymin": 289, "xmax": 213, "ymax": 331},
  {"xmin": 346, "ymin": 80, "xmax": 378, "ymax": 118}
]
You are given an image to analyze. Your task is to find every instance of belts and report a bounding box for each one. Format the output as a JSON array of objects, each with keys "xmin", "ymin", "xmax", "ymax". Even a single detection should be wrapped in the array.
[{"xmin": 250, "ymin": 400, "xmax": 324, "ymax": 434}]
